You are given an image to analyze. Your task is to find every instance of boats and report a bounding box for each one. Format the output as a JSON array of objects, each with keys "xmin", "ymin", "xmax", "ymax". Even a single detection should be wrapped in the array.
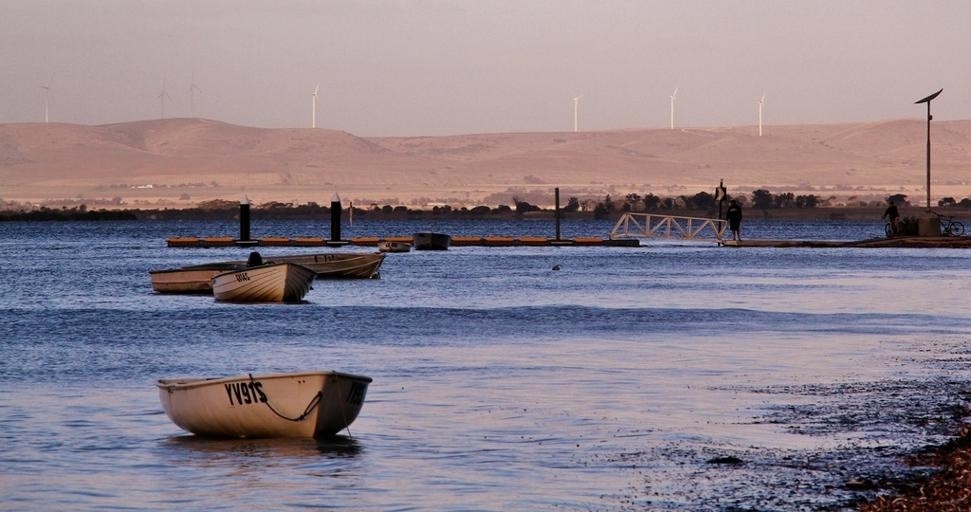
[
  {"xmin": 156, "ymin": 371, "xmax": 373, "ymax": 438},
  {"xmin": 148, "ymin": 252, "xmax": 386, "ymax": 302},
  {"xmin": 379, "ymin": 233, "xmax": 451, "ymax": 251}
]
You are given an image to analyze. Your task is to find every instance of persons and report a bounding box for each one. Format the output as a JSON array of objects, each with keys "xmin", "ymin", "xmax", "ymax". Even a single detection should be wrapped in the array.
[
  {"xmin": 882, "ymin": 201, "xmax": 900, "ymax": 234},
  {"xmin": 726, "ymin": 200, "xmax": 742, "ymax": 241}
]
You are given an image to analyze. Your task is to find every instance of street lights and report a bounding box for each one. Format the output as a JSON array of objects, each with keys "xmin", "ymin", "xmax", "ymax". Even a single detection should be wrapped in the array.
[{"xmin": 914, "ymin": 88, "xmax": 943, "ymax": 237}]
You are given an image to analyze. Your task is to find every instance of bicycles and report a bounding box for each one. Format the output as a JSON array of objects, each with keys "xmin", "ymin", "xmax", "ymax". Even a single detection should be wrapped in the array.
[
  {"xmin": 932, "ymin": 211, "xmax": 964, "ymax": 237},
  {"xmin": 882, "ymin": 214, "xmax": 904, "ymax": 238}
]
